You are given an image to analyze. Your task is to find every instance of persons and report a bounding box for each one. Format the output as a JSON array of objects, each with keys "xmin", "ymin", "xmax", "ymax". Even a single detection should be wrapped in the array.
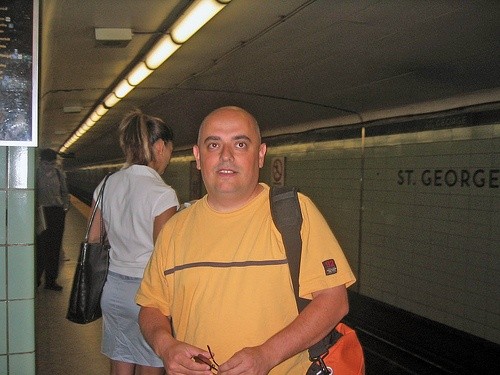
[
  {"xmin": 133, "ymin": 106, "xmax": 357, "ymax": 375},
  {"xmin": 34, "ymin": 148, "xmax": 70, "ymax": 293},
  {"xmin": 88, "ymin": 111, "xmax": 199, "ymax": 375}
]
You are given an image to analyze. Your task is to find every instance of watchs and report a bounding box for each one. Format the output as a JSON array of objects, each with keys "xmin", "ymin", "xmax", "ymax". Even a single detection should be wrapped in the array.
[{"xmin": 182, "ymin": 201, "xmax": 192, "ymax": 209}]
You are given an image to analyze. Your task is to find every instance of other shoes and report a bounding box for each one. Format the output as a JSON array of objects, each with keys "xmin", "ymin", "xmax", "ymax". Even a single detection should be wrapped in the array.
[
  {"xmin": 44, "ymin": 282, "xmax": 64, "ymax": 292},
  {"xmin": 35, "ymin": 274, "xmax": 42, "ymax": 286}
]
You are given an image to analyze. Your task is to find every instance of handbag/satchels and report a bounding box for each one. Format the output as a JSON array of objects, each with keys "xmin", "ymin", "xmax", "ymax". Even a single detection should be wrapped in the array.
[
  {"xmin": 66, "ymin": 170, "xmax": 115, "ymax": 324},
  {"xmin": 269, "ymin": 184, "xmax": 366, "ymax": 375}
]
